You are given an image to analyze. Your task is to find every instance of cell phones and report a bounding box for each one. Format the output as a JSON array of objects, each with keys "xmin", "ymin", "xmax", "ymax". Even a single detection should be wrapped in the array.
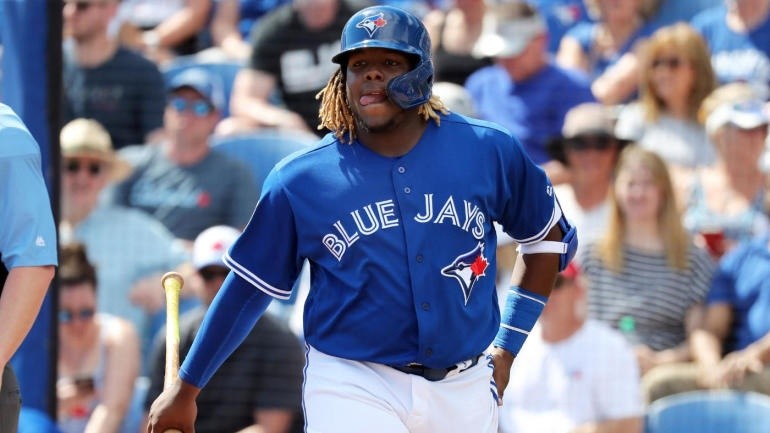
[{"xmin": 75, "ymin": 377, "xmax": 93, "ymax": 388}]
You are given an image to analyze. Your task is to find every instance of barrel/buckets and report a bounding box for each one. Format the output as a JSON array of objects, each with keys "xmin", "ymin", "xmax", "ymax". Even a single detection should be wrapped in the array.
[{"xmin": 648, "ymin": 392, "xmax": 770, "ymax": 433}]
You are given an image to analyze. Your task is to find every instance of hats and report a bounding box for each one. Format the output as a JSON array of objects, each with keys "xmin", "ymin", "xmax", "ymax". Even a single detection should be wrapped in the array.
[
  {"xmin": 59, "ymin": 118, "xmax": 113, "ymax": 161},
  {"xmin": 168, "ymin": 66, "xmax": 224, "ymax": 109},
  {"xmin": 192, "ymin": 224, "xmax": 243, "ymax": 272},
  {"xmin": 470, "ymin": 14, "xmax": 547, "ymax": 60},
  {"xmin": 705, "ymin": 97, "xmax": 769, "ymax": 136},
  {"xmin": 562, "ymin": 101, "xmax": 612, "ymax": 137}
]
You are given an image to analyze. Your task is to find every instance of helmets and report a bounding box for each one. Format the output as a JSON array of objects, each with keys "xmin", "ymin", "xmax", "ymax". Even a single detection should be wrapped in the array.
[{"xmin": 332, "ymin": 6, "xmax": 435, "ymax": 109}]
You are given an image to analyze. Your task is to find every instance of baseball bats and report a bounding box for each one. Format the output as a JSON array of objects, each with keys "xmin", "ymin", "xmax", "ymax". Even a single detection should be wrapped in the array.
[{"xmin": 160, "ymin": 271, "xmax": 185, "ymax": 433}]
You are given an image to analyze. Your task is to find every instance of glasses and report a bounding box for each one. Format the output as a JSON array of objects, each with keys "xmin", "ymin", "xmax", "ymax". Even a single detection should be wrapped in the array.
[
  {"xmin": 71, "ymin": 1, "xmax": 107, "ymax": 11},
  {"xmin": 63, "ymin": 160, "xmax": 103, "ymax": 176},
  {"xmin": 169, "ymin": 95, "xmax": 211, "ymax": 117},
  {"xmin": 56, "ymin": 309, "xmax": 95, "ymax": 325},
  {"xmin": 565, "ymin": 132, "xmax": 611, "ymax": 149},
  {"xmin": 551, "ymin": 274, "xmax": 573, "ymax": 293},
  {"xmin": 649, "ymin": 57, "xmax": 688, "ymax": 69},
  {"xmin": 197, "ymin": 266, "xmax": 232, "ymax": 283},
  {"xmin": 57, "ymin": 275, "xmax": 86, "ymax": 289}
]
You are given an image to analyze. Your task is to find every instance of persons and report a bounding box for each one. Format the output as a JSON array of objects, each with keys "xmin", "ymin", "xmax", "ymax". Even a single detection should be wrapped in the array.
[
  {"xmin": 0, "ymin": 0, "xmax": 770, "ymax": 433},
  {"xmin": 147, "ymin": 6, "xmax": 578, "ymax": 433}
]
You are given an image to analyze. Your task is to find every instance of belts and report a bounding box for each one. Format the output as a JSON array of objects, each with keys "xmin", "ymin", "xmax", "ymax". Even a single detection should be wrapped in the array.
[{"xmin": 385, "ymin": 355, "xmax": 481, "ymax": 381}]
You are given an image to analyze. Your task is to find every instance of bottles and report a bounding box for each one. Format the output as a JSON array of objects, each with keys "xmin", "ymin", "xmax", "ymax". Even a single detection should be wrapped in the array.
[{"xmin": 618, "ymin": 318, "xmax": 639, "ymax": 345}]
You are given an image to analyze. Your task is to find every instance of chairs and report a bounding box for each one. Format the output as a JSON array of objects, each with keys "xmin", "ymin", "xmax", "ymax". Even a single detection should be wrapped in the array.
[
  {"xmin": 645, "ymin": 388, "xmax": 770, "ymax": 433},
  {"xmin": 161, "ymin": 62, "xmax": 313, "ymax": 198}
]
